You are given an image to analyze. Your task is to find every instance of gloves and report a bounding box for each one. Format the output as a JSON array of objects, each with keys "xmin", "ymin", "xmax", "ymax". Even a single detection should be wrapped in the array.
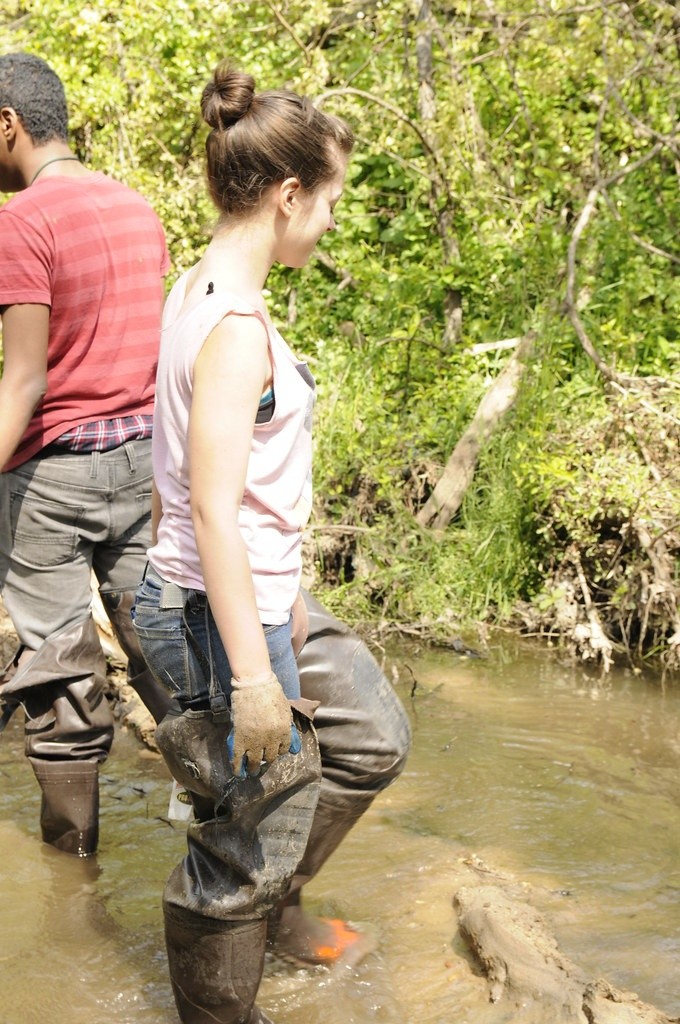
[{"xmin": 229, "ymin": 672, "xmax": 302, "ymax": 779}]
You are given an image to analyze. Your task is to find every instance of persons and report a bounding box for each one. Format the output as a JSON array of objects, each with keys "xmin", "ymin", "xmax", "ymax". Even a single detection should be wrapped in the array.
[
  {"xmin": 131, "ymin": 61, "xmax": 411, "ymax": 1024},
  {"xmin": 0, "ymin": 49, "xmax": 200, "ymax": 854}
]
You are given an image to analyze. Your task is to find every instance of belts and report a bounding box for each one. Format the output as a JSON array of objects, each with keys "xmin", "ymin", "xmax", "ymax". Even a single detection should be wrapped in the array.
[{"xmin": 145, "ymin": 567, "xmax": 187, "ymax": 608}]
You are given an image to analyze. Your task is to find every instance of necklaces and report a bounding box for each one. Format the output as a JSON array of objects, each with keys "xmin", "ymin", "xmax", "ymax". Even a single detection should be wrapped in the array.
[{"xmin": 29, "ymin": 152, "xmax": 79, "ymax": 186}]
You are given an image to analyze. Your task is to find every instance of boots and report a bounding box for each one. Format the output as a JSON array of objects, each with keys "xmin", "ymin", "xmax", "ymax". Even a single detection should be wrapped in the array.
[
  {"xmin": 96, "ymin": 578, "xmax": 174, "ymax": 726},
  {"xmin": 157, "ymin": 690, "xmax": 322, "ymax": 1024},
  {"xmin": 3, "ymin": 612, "xmax": 99, "ymax": 857},
  {"xmin": 262, "ymin": 586, "xmax": 413, "ymax": 962}
]
